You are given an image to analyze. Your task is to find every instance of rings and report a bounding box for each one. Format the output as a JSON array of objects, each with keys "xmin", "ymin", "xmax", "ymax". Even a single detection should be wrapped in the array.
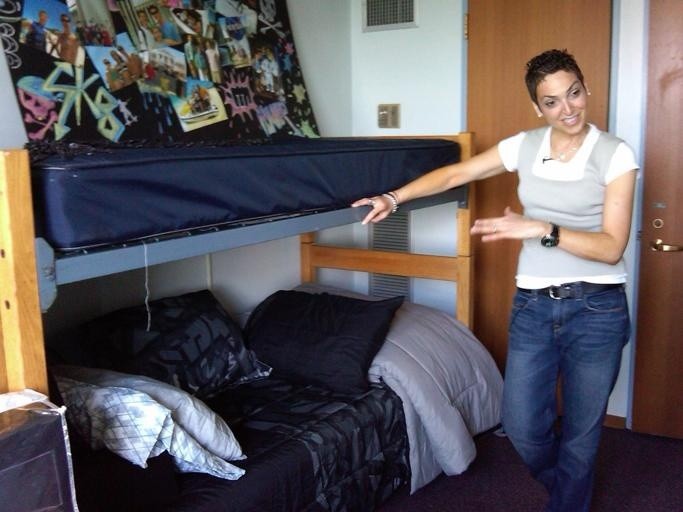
[{"xmin": 368, "ymin": 199, "xmax": 372, "ymax": 205}]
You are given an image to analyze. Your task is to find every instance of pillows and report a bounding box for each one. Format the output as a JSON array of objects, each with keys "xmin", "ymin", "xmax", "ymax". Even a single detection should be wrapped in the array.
[
  {"xmin": 94, "ymin": 288, "xmax": 273, "ymax": 402},
  {"xmin": 52, "ymin": 370, "xmax": 250, "ymax": 485},
  {"xmin": 244, "ymin": 289, "xmax": 407, "ymax": 396}
]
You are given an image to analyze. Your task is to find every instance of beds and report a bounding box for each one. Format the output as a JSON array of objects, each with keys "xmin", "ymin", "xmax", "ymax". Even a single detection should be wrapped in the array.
[{"xmin": 1, "ymin": 134, "xmax": 480, "ymax": 508}]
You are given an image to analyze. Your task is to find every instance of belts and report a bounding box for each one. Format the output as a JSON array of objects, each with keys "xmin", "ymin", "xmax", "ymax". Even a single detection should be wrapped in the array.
[{"xmin": 514, "ymin": 279, "xmax": 622, "ymax": 300}]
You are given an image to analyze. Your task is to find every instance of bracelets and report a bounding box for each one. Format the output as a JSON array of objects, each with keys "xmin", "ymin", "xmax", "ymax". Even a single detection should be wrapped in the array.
[{"xmin": 382, "ymin": 192, "xmax": 399, "ymax": 213}]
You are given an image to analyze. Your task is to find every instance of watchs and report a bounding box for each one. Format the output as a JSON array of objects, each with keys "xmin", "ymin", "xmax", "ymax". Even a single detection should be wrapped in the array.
[{"xmin": 541, "ymin": 222, "xmax": 559, "ymax": 248}]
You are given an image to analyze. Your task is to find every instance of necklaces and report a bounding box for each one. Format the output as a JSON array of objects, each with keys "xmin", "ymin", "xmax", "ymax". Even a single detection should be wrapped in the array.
[{"xmin": 551, "ymin": 145, "xmax": 577, "ymax": 160}]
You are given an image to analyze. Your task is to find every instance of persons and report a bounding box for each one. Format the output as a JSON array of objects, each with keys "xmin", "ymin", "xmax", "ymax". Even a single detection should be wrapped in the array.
[
  {"xmin": 21, "ymin": 6, "xmax": 279, "ymax": 115},
  {"xmin": 350, "ymin": 48, "xmax": 640, "ymax": 512}
]
